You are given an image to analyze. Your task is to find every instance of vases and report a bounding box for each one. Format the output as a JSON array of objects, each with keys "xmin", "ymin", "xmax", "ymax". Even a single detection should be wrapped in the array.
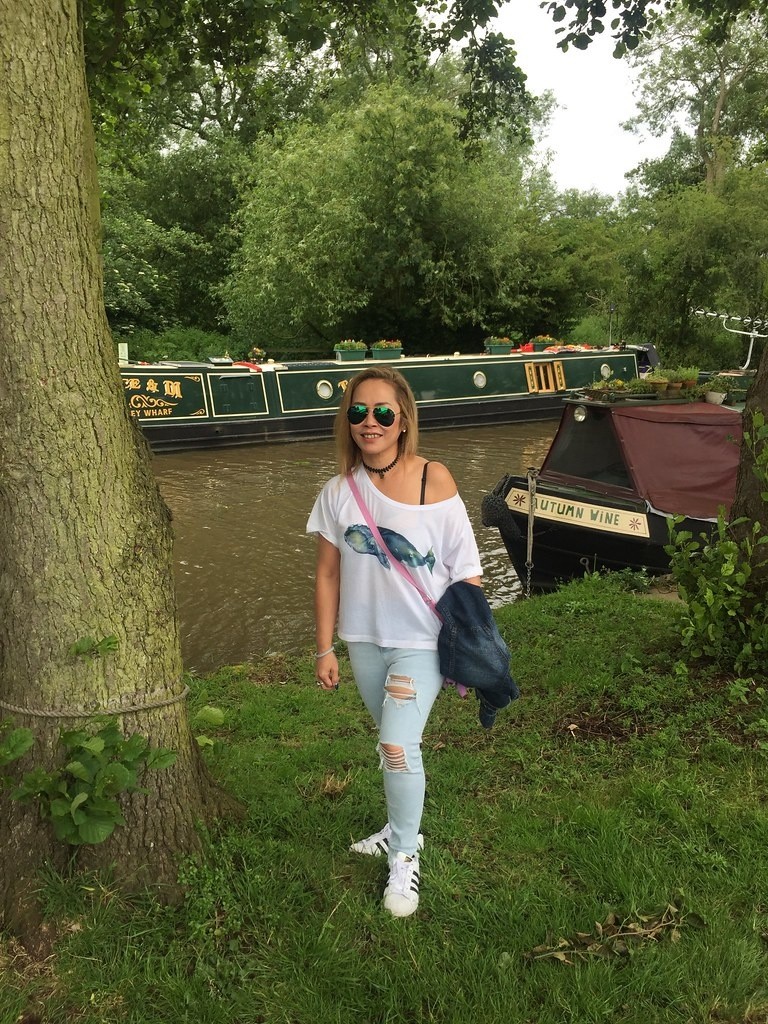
[
  {"xmin": 484, "ymin": 345, "xmax": 513, "ymax": 355},
  {"xmin": 533, "ymin": 343, "xmax": 554, "ymax": 352},
  {"xmin": 250, "ymin": 358, "xmax": 263, "ymax": 364},
  {"xmin": 370, "ymin": 348, "xmax": 403, "ymax": 360},
  {"xmin": 334, "ymin": 350, "xmax": 366, "ymax": 361}
]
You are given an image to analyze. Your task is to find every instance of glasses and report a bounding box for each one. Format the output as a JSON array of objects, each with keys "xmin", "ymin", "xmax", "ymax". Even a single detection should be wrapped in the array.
[{"xmin": 347, "ymin": 405, "xmax": 396, "ymax": 430}]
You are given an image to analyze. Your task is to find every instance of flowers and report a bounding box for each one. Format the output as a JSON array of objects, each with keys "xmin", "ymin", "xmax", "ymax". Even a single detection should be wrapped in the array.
[
  {"xmin": 334, "ymin": 339, "xmax": 367, "ymax": 350},
  {"xmin": 370, "ymin": 339, "xmax": 401, "ymax": 349},
  {"xmin": 484, "ymin": 336, "xmax": 513, "ymax": 345},
  {"xmin": 247, "ymin": 348, "xmax": 266, "ymax": 360},
  {"xmin": 529, "ymin": 335, "xmax": 557, "ymax": 343}
]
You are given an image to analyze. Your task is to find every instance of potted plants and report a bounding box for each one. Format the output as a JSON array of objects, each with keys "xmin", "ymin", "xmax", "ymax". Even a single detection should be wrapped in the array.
[
  {"xmin": 676, "ymin": 364, "xmax": 700, "ymax": 389},
  {"xmin": 685, "ymin": 375, "xmax": 740, "ymax": 406},
  {"xmin": 643, "ymin": 365, "xmax": 669, "ymax": 392},
  {"xmin": 661, "ymin": 369, "xmax": 685, "ymax": 396}
]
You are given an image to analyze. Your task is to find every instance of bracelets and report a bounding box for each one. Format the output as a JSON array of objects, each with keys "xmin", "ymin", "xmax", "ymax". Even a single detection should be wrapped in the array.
[{"xmin": 314, "ymin": 645, "xmax": 335, "ymax": 659}]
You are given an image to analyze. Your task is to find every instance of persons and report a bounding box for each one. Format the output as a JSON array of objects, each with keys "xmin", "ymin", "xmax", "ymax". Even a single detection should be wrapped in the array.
[{"xmin": 305, "ymin": 368, "xmax": 484, "ymax": 919}]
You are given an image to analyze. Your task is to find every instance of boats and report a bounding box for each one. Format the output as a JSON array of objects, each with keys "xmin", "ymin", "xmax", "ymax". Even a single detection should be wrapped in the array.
[
  {"xmin": 484, "ymin": 390, "xmax": 744, "ymax": 596},
  {"xmin": 116, "ymin": 339, "xmax": 643, "ymax": 454}
]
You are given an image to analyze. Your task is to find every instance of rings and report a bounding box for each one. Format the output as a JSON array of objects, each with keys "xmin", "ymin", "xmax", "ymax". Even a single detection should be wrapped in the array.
[{"xmin": 317, "ymin": 682, "xmax": 323, "ymax": 685}]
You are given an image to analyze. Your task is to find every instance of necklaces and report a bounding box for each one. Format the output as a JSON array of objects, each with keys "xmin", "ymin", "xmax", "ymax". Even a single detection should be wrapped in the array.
[{"xmin": 361, "ymin": 450, "xmax": 400, "ymax": 479}]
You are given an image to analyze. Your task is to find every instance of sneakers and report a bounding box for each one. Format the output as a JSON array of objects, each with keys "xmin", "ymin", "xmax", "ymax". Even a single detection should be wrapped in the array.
[
  {"xmin": 383, "ymin": 849, "xmax": 420, "ymax": 918},
  {"xmin": 349, "ymin": 823, "xmax": 425, "ymax": 858}
]
